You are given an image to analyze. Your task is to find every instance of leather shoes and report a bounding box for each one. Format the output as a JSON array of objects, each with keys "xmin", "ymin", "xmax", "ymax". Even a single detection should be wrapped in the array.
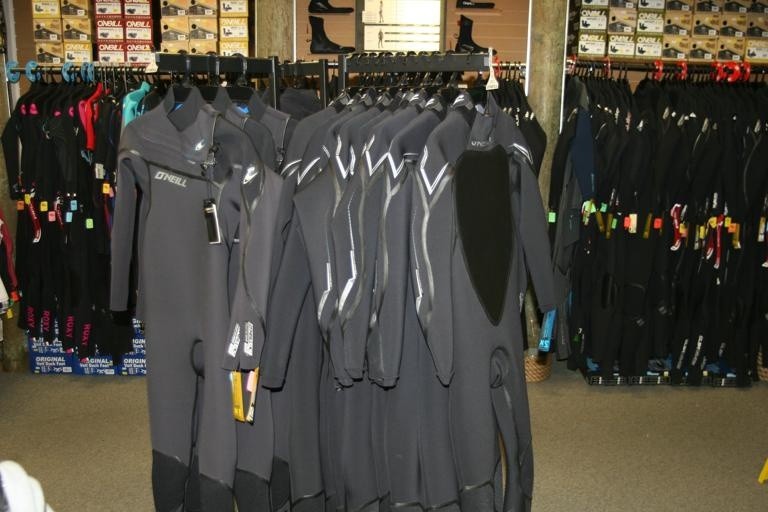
[
  {"xmin": 456, "ymin": 0, "xmax": 495, "ymax": 8},
  {"xmin": 308, "ymin": 0, "xmax": 354, "ymax": 13}
]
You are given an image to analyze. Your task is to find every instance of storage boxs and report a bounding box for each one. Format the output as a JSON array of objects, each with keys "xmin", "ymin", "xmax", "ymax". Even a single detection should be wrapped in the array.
[
  {"xmin": 160, "ymin": 40, "xmax": 189, "ymax": 55},
  {"xmin": 64, "ymin": 43, "xmax": 93, "ymax": 64},
  {"xmin": 160, "ymin": 16, "xmax": 189, "ymax": 41},
  {"xmin": 126, "ymin": 44, "xmax": 155, "ymax": 67},
  {"xmin": 37, "ymin": 42, "xmax": 63, "ymax": 64},
  {"xmin": 97, "ymin": 43, "xmax": 125, "ymax": 66},
  {"xmin": 189, "ymin": 41, "xmax": 216, "ymax": 55},
  {"xmin": 160, "ymin": 0, "xmax": 188, "ymax": 15},
  {"xmin": 96, "ymin": 18, "xmax": 124, "ymax": 42},
  {"xmin": 93, "ymin": 1, "xmax": 122, "ymax": 18},
  {"xmin": 29, "ymin": 0, "xmax": 60, "ymax": 18},
  {"xmin": 62, "ymin": 18, "xmax": 92, "ymax": 42},
  {"xmin": 126, "ymin": 18, "xmax": 153, "ymax": 43},
  {"xmin": 61, "ymin": 0, "xmax": 88, "ymax": 17},
  {"xmin": 189, "ymin": 0, "xmax": 218, "ymax": 16},
  {"xmin": 188, "ymin": 17, "xmax": 216, "ymax": 38},
  {"xmin": 219, "ymin": 1, "xmax": 248, "ymax": 17},
  {"xmin": 218, "ymin": 41, "xmax": 249, "ymax": 58},
  {"xmin": 219, "ymin": 18, "xmax": 249, "ymax": 41},
  {"xmin": 34, "ymin": 19, "xmax": 63, "ymax": 40},
  {"xmin": 124, "ymin": 1, "xmax": 152, "ymax": 15},
  {"xmin": 568, "ymin": 0, "xmax": 764, "ymax": 59}
]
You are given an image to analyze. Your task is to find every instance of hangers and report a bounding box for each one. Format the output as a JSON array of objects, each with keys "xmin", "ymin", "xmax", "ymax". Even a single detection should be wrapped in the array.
[
  {"xmin": 563, "ymin": 58, "xmax": 764, "ymax": 100},
  {"xmin": 3, "ymin": 58, "xmax": 529, "ymax": 146}
]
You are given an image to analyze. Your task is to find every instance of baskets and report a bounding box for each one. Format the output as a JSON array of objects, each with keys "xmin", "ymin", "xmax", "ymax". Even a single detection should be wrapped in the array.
[{"xmin": 524, "ymin": 355, "xmax": 550, "ymax": 384}]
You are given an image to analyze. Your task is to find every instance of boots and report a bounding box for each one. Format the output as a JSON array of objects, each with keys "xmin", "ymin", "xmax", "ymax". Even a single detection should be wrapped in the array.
[
  {"xmin": 308, "ymin": 16, "xmax": 355, "ymax": 53},
  {"xmin": 456, "ymin": 14, "xmax": 497, "ymax": 56}
]
